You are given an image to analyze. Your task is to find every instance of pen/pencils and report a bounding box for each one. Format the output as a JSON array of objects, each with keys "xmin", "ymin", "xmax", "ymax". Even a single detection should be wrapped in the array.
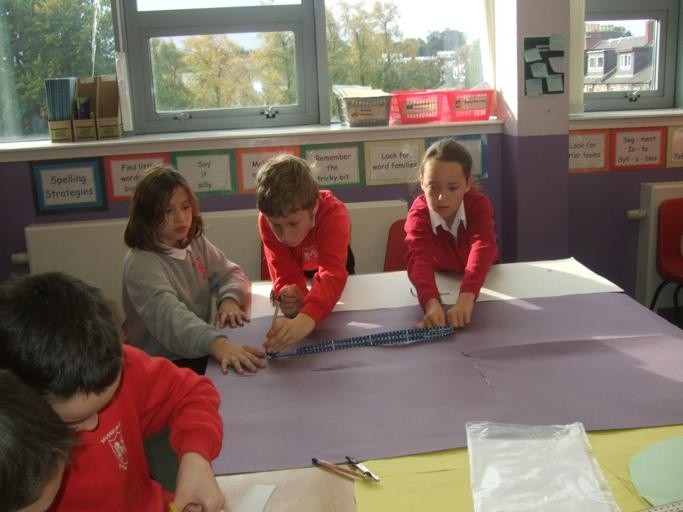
[
  {"xmin": 271, "ymin": 294, "xmax": 283, "ymax": 328},
  {"xmin": 312, "ymin": 457, "xmax": 366, "ymax": 481}
]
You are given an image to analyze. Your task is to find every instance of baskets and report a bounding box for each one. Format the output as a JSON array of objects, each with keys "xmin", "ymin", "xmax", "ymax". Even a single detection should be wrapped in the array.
[
  {"xmin": 338, "ymin": 96, "xmax": 391, "ymax": 127},
  {"xmin": 442, "ymin": 90, "xmax": 494, "ymax": 121},
  {"xmin": 389, "ymin": 89, "xmax": 442, "ymax": 123}
]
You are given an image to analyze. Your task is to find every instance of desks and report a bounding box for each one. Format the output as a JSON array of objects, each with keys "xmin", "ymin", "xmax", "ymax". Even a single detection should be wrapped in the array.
[{"xmin": 203, "ymin": 257, "xmax": 683, "ymax": 511}]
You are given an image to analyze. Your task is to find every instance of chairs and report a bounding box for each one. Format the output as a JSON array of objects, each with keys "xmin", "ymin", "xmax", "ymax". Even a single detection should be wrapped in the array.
[{"xmin": 651, "ymin": 198, "xmax": 682, "ymax": 324}]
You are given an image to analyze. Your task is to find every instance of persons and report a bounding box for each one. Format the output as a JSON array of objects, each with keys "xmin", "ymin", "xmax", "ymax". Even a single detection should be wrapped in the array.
[
  {"xmin": 254, "ymin": 151, "xmax": 355, "ymax": 355},
  {"xmin": 403, "ymin": 139, "xmax": 500, "ymax": 330},
  {"xmin": 121, "ymin": 161, "xmax": 268, "ymax": 374},
  {"xmin": 1, "ymin": 271, "xmax": 226, "ymax": 511},
  {"xmin": 0, "ymin": 369, "xmax": 75, "ymax": 511}
]
